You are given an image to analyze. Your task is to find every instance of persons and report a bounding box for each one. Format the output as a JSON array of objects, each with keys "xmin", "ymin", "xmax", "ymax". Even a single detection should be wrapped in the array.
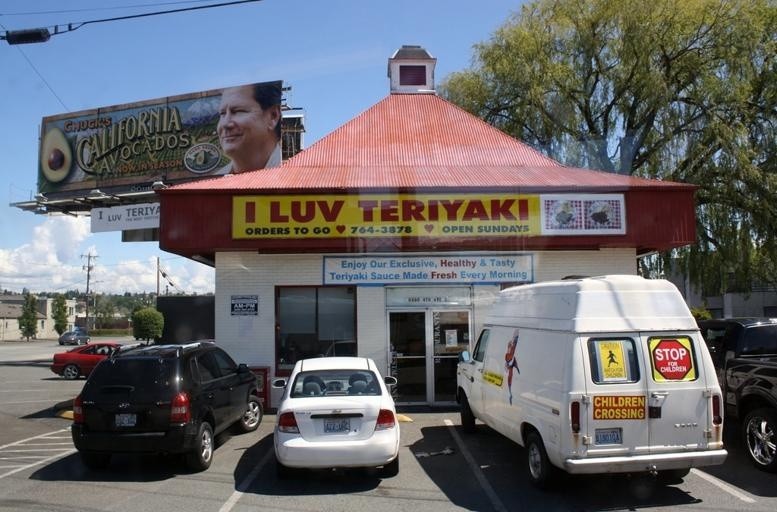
[{"xmin": 217, "ymin": 79, "xmax": 283, "ymax": 175}]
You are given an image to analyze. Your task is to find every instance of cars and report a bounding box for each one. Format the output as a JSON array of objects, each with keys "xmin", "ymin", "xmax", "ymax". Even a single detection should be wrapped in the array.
[
  {"xmin": 319, "ymin": 340, "xmax": 356, "ymax": 358},
  {"xmin": 49, "ymin": 342, "xmax": 124, "ymax": 380},
  {"xmin": 269, "ymin": 355, "xmax": 400, "ymax": 479},
  {"xmin": 58, "ymin": 331, "xmax": 89, "ymax": 346}
]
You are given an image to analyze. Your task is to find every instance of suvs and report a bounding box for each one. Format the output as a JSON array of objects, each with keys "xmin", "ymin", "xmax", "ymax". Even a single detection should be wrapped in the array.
[
  {"xmin": 71, "ymin": 342, "xmax": 263, "ymax": 472},
  {"xmin": 696, "ymin": 317, "xmax": 776, "ymax": 472}
]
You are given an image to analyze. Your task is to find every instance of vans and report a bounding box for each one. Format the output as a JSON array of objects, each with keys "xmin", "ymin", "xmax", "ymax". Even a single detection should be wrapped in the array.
[{"xmin": 453, "ymin": 273, "xmax": 727, "ymax": 484}]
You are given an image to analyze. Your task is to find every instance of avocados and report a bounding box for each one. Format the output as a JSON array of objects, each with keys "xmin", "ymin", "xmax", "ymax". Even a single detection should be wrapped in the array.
[
  {"xmin": 196, "ymin": 151, "xmax": 207, "ymax": 165},
  {"xmin": 41, "ymin": 128, "xmax": 72, "ymax": 183}
]
